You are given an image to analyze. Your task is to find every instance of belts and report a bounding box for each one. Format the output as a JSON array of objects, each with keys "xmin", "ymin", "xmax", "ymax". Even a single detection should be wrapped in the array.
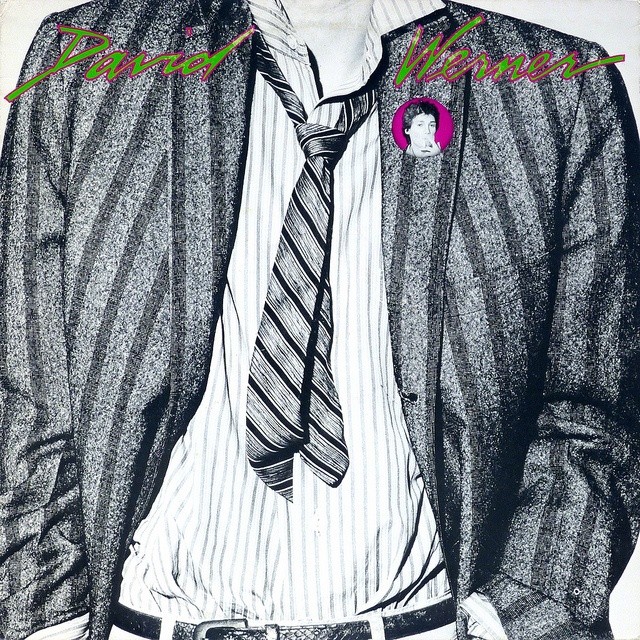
[{"xmin": 110, "ymin": 598, "xmax": 454, "ymax": 640}]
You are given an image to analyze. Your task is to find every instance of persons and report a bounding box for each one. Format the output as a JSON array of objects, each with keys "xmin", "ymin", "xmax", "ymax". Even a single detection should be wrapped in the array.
[
  {"xmin": 1, "ymin": 0, "xmax": 640, "ymax": 640},
  {"xmin": 399, "ymin": 98, "xmax": 442, "ymax": 158}
]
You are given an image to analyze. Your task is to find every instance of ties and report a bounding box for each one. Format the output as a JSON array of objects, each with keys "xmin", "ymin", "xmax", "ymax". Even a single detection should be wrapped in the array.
[{"xmin": 246, "ymin": 33, "xmax": 376, "ymax": 501}]
